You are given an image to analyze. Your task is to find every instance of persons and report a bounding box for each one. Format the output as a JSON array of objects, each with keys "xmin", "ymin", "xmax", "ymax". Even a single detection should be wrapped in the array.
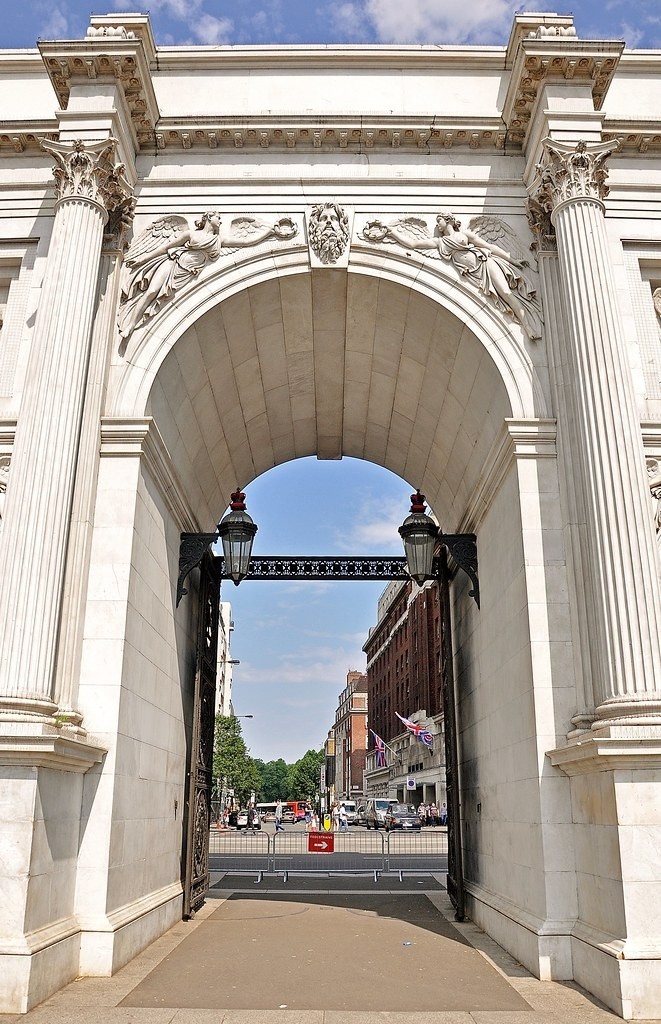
[
  {"xmin": 330, "ymin": 802, "xmax": 349, "ymax": 833},
  {"xmin": 119, "ymin": 211, "xmax": 277, "ymax": 338},
  {"xmin": 241, "ymin": 803, "xmax": 255, "ymax": 835},
  {"xmin": 417, "ymin": 801, "xmax": 448, "ymax": 827},
  {"xmin": 308, "ymin": 202, "xmax": 348, "ymax": 264},
  {"xmin": 304, "ymin": 799, "xmax": 314, "ymax": 832},
  {"xmin": 217, "ymin": 802, "xmax": 239, "ymax": 829},
  {"xmin": 384, "ymin": 211, "xmax": 539, "ymax": 338},
  {"xmin": 275, "ymin": 799, "xmax": 285, "ymax": 831}
]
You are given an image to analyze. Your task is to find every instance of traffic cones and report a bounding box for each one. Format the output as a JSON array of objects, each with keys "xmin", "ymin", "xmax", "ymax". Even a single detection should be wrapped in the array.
[
  {"xmin": 215, "ymin": 818, "xmax": 223, "ymax": 829},
  {"xmin": 310, "ymin": 815, "xmax": 318, "ymax": 831}
]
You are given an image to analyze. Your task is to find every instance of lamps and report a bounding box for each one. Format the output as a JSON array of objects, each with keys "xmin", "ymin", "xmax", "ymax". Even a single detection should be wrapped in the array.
[
  {"xmin": 399, "ymin": 490, "xmax": 480, "ymax": 611},
  {"xmin": 175, "ymin": 487, "xmax": 258, "ymax": 609}
]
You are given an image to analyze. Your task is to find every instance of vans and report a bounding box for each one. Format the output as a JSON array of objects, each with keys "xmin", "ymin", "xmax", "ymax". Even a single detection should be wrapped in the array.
[
  {"xmin": 338, "ymin": 801, "xmax": 366, "ymax": 826},
  {"xmin": 364, "ymin": 798, "xmax": 400, "ymax": 829}
]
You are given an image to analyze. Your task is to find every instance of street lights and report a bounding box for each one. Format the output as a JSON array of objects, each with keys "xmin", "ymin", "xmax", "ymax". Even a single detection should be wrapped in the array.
[{"xmin": 231, "ymin": 715, "xmax": 253, "ymax": 811}]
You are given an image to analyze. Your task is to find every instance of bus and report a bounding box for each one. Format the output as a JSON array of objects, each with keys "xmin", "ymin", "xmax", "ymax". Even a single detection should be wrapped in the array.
[{"xmin": 256, "ymin": 801, "xmax": 306, "ymax": 822}]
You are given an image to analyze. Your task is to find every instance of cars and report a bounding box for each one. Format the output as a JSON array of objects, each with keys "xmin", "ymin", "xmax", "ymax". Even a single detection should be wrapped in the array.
[
  {"xmin": 236, "ymin": 810, "xmax": 297, "ymax": 830},
  {"xmin": 385, "ymin": 803, "xmax": 422, "ymax": 834}
]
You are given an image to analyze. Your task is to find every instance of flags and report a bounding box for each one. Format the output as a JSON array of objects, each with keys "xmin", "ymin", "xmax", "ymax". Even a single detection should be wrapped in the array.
[
  {"xmin": 394, "ymin": 712, "xmax": 434, "ymax": 747},
  {"xmin": 369, "ymin": 729, "xmax": 386, "ymax": 767}
]
